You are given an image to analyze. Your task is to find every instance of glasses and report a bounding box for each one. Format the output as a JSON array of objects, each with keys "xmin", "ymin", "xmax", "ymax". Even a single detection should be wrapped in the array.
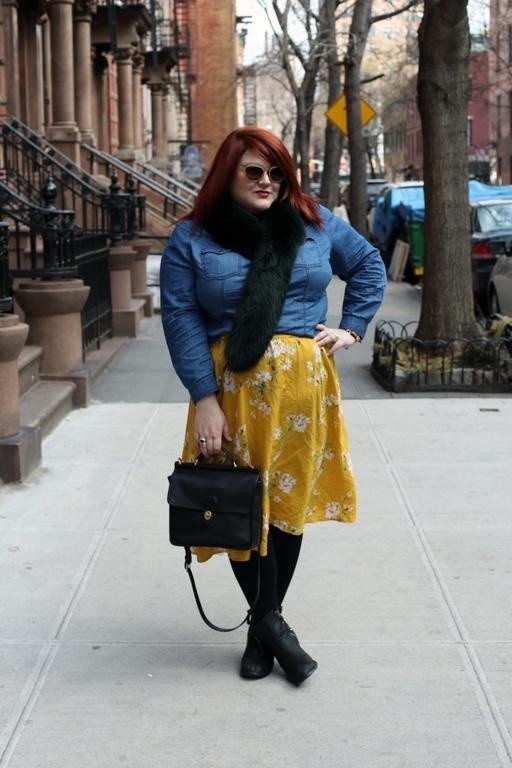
[{"xmin": 239, "ymin": 162, "xmax": 285, "ymax": 184}]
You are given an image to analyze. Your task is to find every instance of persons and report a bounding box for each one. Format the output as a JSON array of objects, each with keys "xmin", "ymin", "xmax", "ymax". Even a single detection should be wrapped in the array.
[
  {"xmin": 160, "ymin": 127, "xmax": 387, "ymax": 688},
  {"xmin": 404, "ymin": 164, "xmax": 420, "ymax": 181}
]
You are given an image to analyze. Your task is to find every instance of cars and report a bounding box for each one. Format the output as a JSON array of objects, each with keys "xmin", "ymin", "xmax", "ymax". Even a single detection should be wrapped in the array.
[{"xmin": 297, "ymin": 170, "xmax": 510, "ymax": 356}]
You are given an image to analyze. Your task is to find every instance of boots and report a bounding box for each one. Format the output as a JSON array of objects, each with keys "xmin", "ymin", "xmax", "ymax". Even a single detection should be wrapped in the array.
[
  {"xmin": 239, "ymin": 609, "xmax": 274, "ymax": 681},
  {"xmin": 267, "ymin": 614, "xmax": 319, "ymax": 688}
]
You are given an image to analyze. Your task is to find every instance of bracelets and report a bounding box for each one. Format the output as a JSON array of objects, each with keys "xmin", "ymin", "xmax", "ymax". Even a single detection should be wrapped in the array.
[{"xmin": 346, "ymin": 329, "xmax": 360, "ymax": 342}]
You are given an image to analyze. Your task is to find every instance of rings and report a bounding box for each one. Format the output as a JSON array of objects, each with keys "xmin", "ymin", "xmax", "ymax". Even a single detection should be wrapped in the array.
[{"xmin": 199, "ymin": 437, "xmax": 206, "ymax": 444}]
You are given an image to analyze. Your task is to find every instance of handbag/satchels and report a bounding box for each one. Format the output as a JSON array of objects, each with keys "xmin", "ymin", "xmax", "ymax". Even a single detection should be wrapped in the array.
[{"xmin": 166, "ymin": 445, "xmax": 263, "ymax": 550}]
